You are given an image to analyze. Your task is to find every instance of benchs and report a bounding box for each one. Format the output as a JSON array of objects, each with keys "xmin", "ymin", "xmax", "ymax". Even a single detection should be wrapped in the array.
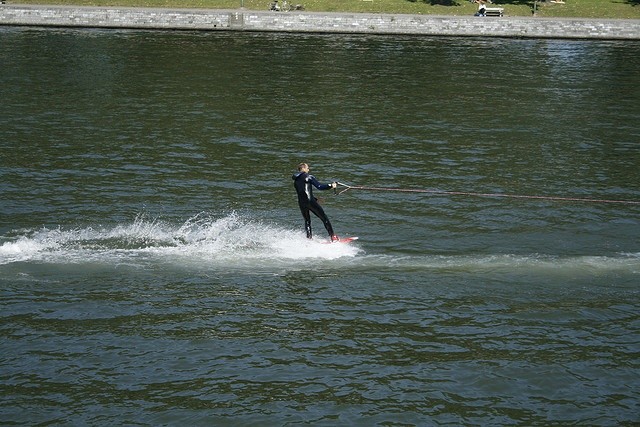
[{"xmin": 478, "ymin": 8, "xmax": 504, "ymax": 17}]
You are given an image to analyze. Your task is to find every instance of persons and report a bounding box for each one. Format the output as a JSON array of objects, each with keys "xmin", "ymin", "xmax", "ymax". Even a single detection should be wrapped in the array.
[
  {"xmin": 475, "ymin": 0, "xmax": 486, "ymax": 17},
  {"xmin": 291, "ymin": 161, "xmax": 341, "ymax": 241}
]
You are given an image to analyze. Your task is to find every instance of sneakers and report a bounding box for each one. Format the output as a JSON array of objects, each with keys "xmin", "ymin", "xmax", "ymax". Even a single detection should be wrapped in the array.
[{"xmin": 331, "ymin": 235, "xmax": 339, "ymax": 242}]
